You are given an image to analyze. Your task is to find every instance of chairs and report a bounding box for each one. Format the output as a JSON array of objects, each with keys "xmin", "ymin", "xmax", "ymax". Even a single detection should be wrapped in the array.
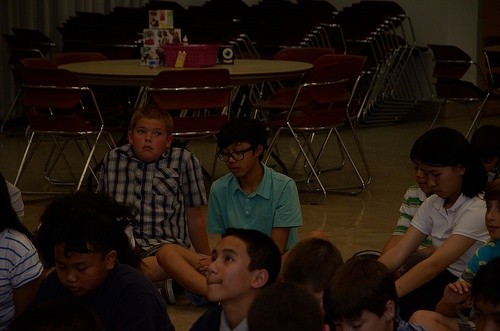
[{"xmin": 0, "ymin": 1, "xmax": 500, "ymax": 205}]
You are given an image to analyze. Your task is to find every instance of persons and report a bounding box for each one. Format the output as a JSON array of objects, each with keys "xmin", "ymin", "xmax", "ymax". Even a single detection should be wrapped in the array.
[
  {"xmin": 2, "ymin": 190, "xmax": 176, "ymax": 331},
  {"xmin": 322, "ymin": 256, "xmax": 424, "ymax": 331},
  {"xmin": 150, "ymin": 12, "xmax": 159, "ymax": 27},
  {"xmin": 378, "ymin": 123, "xmax": 500, "ymax": 331},
  {"xmin": 0, "ymin": 172, "xmax": 44, "ymax": 331},
  {"xmin": 157, "ymin": 117, "xmax": 303, "ymax": 301},
  {"xmin": 100, "ymin": 106, "xmax": 212, "ymax": 303},
  {"xmin": 6, "ymin": 181, "xmax": 24, "ymax": 222},
  {"xmin": 247, "ymin": 282, "xmax": 329, "ymax": 331},
  {"xmin": 188, "ymin": 228, "xmax": 282, "ymax": 331},
  {"xmin": 282, "ymin": 236, "xmax": 343, "ymax": 299},
  {"xmin": 158, "ymin": 30, "xmax": 169, "ymax": 46},
  {"xmin": 170, "ymin": 30, "xmax": 181, "ymax": 43}
]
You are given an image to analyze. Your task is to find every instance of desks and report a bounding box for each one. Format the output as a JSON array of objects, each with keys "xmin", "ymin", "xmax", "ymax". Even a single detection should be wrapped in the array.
[{"xmin": 59, "ymin": 58, "xmax": 313, "ymax": 184}]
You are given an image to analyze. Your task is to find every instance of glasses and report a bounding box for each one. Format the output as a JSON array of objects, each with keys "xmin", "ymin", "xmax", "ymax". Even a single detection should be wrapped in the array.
[{"xmin": 216, "ymin": 145, "xmax": 256, "ymax": 162}]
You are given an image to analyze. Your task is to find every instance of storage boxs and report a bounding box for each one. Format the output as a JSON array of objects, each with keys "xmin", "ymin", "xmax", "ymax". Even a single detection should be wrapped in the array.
[
  {"xmin": 217, "ymin": 45, "xmax": 235, "ymax": 64},
  {"xmin": 140, "ymin": 47, "xmax": 166, "ymax": 66},
  {"xmin": 143, "ymin": 28, "xmax": 181, "ymax": 47},
  {"xmin": 149, "ymin": 9, "xmax": 174, "ymax": 29}
]
